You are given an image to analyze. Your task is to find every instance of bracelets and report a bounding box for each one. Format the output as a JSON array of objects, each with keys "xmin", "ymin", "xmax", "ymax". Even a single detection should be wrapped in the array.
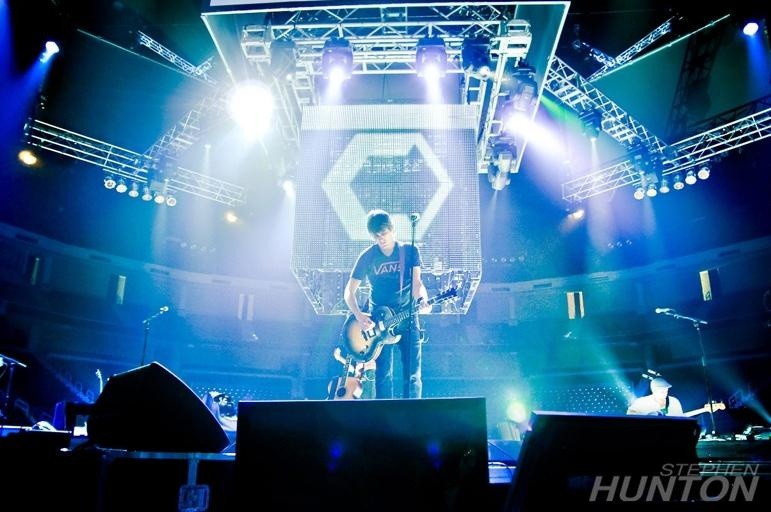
[{"xmin": 416, "ymin": 297, "xmax": 424, "ymax": 304}]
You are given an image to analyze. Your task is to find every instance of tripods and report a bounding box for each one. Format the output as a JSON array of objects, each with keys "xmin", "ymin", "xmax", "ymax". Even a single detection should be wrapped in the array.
[{"xmin": 665, "ymin": 311, "xmax": 733, "ymax": 443}]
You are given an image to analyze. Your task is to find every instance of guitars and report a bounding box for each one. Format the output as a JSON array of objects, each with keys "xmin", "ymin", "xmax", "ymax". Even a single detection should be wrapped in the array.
[
  {"xmin": 326, "ymin": 353, "xmax": 364, "ymax": 400},
  {"xmin": 343, "ymin": 288, "xmax": 458, "ymax": 363},
  {"xmin": 648, "ymin": 400, "xmax": 726, "ymax": 417}
]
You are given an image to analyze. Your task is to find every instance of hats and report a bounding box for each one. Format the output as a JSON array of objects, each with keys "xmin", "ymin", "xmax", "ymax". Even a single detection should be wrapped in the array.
[{"xmin": 650, "ymin": 378, "xmax": 672, "ymax": 388}]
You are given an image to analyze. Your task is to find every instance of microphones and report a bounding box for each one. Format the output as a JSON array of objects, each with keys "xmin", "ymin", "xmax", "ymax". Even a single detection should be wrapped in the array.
[
  {"xmin": 409, "ymin": 212, "xmax": 421, "ymax": 225},
  {"xmin": 159, "ymin": 305, "xmax": 170, "ymax": 315},
  {"xmin": 655, "ymin": 307, "xmax": 675, "ymax": 313}
]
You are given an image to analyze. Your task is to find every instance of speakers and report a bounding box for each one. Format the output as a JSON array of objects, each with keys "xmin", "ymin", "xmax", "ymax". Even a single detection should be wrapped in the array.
[
  {"xmin": 511, "ymin": 411, "xmax": 701, "ymax": 485},
  {"xmin": 87, "ymin": 361, "xmax": 230, "ymax": 453},
  {"xmin": 235, "ymin": 397, "xmax": 488, "ymax": 482}
]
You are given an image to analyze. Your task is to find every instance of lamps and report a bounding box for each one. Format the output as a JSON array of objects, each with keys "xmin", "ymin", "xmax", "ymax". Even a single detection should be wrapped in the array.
[
  {"xmin": 633, "ymin": 157, "xmax": 713, "ymax": 201},
  {"xmin": 487, "ymin": 70, "xmax": 538, "ymax": 191},
  {"xmin": 102, "ymin": 173, "xmax": 178, "ymax": 208},
  {"xmin": 319, "ymin": 32, "xmax": 493, "ymax": 84},
  {"xmin": 579, "ymin": 106, "xmax": 605, "ymax": 144}
]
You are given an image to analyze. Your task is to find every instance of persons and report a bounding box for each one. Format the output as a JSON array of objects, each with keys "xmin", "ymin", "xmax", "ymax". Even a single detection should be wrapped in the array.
[
  {"xmin": 344, "ymin": 209, "xmax": 432, "ymax": 400},
  {"xmin": 626, "ymin": 378, "xmax": 684, "ymax": 418}
]
